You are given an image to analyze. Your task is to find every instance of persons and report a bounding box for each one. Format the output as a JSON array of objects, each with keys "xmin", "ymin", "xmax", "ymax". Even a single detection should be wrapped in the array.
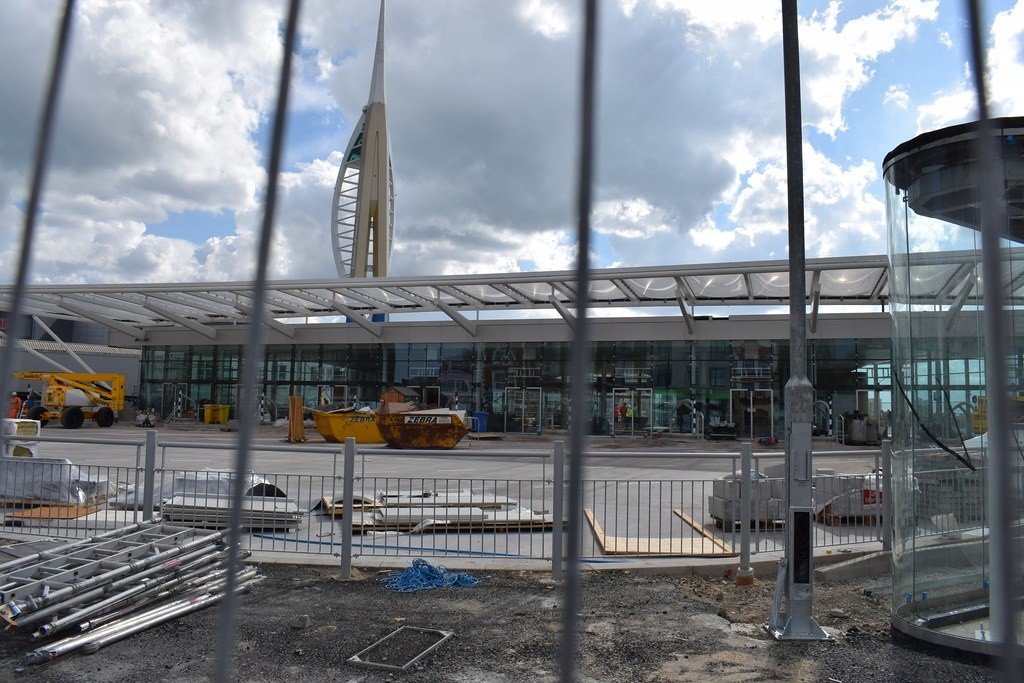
[
  {"xmin": 177, "ymin": 411, "xmax": 181, "ymax": 421},
  {"xmin": 136, "ymin": 409, "xmax": 158, "ymax": 415},
  {"xmin": 18, "ymin": 395, "xmax": 36, "ymax": 419},
  {"xmin": 407, "ymin": 403, "xmax": 415, "ymax": 411},
  {"xmin": 614, "ymin": 402, "xmax": 629, "ymax": 423},
  {"xmin": 970, "ymin": 396, "xmax": 977, "ymax": 413},
  {"xmin": 7, "ymin": 392, "xmax": 21, "ymax": 419},
  {"xmin": 1011, "ymin": 383, "xmax": 1018, "ymax": 398}
]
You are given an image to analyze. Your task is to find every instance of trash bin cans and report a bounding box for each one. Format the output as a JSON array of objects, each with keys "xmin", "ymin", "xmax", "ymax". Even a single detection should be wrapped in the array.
[
  {"xmin": 473, "ymin": 411, "xmax": 490, "ymax": 431},
  {"xmin": 204, "ymin": 404, "xmax": 231, "ymax": 424},
  {"xmin": 196, "ymin": 398, "xmax": 211, "ymax": 423}
]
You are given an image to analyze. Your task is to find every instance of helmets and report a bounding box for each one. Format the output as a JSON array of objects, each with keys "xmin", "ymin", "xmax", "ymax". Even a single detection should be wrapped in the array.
[{"xmin": 12, "ymin": 392, "xmax": 17, "ymax": 395}]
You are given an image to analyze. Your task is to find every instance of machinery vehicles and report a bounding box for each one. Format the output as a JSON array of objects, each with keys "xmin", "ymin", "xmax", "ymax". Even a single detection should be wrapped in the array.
[{"xmin": 9, "ymin": 371, "xmax": 125, "ymax": 431}]
